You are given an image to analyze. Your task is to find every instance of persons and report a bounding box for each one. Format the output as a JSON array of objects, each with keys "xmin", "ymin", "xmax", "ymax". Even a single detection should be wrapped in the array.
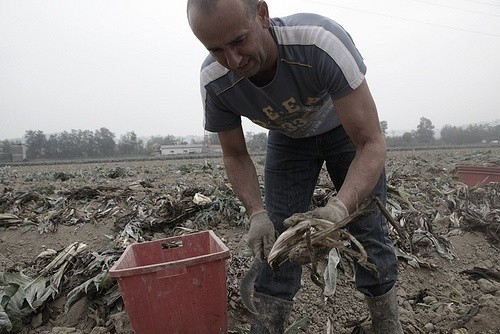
[{"xmin": 187, "ymin": 0, "xmax": 404, "ymax": 334}]
